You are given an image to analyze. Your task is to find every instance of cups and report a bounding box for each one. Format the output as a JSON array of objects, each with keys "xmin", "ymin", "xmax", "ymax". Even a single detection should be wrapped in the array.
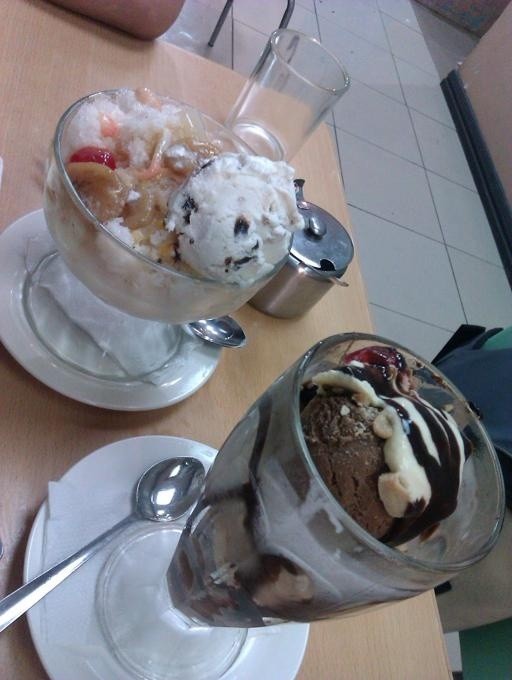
[
  {"xmin": 224, "ymin": 27, "xmax": 352, "ymax": 168},
  {"xmin": 245, "ymin": 173, "xmax": 356, "ymax": 322}
]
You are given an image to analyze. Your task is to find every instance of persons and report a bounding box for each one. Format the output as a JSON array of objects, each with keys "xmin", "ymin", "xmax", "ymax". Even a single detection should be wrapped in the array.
[{"xmin": 46, "ymin": 1, "xmax": 189, "ymax": 41}]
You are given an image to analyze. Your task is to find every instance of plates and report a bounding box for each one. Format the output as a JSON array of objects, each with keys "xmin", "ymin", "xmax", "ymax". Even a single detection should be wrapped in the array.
[
  {"xmin": 0, "ymin": 209, "xmax": 230, "ymax": 412},
  {"xmin": 91, "ymin": 332, "xmax": 506, "ymax": 680},
  {"xmin": 20, "ymin": 433, "xmax": 314, "ymax": 679}
]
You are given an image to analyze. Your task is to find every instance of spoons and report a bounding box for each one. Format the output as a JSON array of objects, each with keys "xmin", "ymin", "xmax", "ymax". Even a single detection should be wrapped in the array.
[
  {"xmin": 1, "ymin": 455, "xmax": 206, "ymax": 633},
  {"xmin": 190, "ymin": 315, "xmax": 250, "ymax": 349}
]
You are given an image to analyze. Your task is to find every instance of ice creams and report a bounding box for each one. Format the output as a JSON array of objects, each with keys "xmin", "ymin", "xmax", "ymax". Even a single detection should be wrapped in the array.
[
  {"xmin": 163, "ymin": 342, "xmax": 468, "ymax": 630},
  {"xmin": 41, "ymin": 83, "xmax": 307, "ymax": 327}
]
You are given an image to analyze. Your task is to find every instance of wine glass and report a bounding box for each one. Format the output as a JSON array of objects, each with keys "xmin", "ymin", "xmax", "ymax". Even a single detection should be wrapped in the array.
[{"xmin": 22, "ymin": 88, "xmax": 296, "ymax": 381}]
